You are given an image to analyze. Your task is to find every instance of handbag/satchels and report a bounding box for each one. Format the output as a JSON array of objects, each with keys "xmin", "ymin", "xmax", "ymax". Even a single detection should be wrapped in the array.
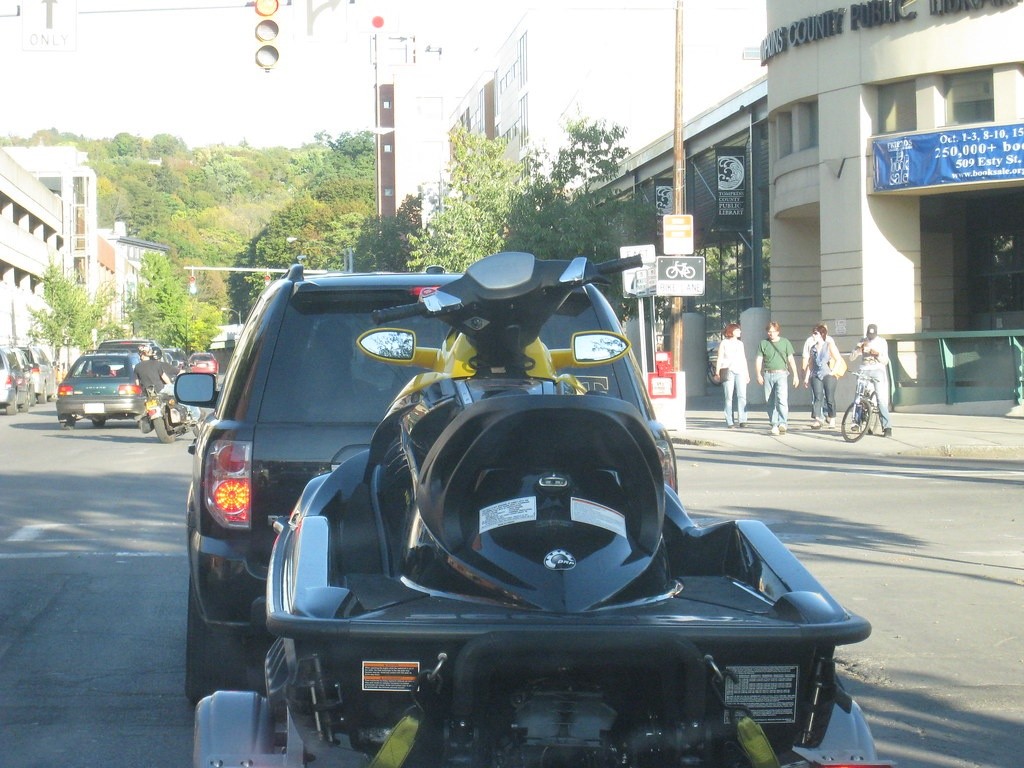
[
  {"xmin": 827, "ymin": 335, "xmax": 847, "ymax": 379},
  {"xmin": 719, "ymin": 367, "xmax": 730, "ymax": 383}
]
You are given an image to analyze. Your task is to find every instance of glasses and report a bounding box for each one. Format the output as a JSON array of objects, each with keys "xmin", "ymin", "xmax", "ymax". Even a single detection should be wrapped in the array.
[
  {"xmin": 813, "ymin": 331, "xmax": 820, "ymax": 335},
  {"xmin": 768, "ymin": 330, "xmax": 778, "ymax": 333}
]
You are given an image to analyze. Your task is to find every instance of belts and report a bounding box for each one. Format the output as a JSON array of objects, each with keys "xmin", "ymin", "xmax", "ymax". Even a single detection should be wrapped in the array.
[{"xmin": 765, "ymin": 370, "xmax": 787, "ymax": 374}]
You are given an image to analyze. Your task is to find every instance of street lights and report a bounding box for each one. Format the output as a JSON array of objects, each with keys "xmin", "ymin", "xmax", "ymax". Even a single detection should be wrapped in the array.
[{"xmin": 286, "ymin": 236, "xmax": 355, "ymax": 273}]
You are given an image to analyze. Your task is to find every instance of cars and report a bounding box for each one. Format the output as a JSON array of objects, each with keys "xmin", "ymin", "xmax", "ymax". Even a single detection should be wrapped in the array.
[
  {"xmin": 0, "ymin": 345, "xmax": 58, "ymax": 416},
  {"xmin": 96, "ymin": 340, "xmax": 218, "ymax": 381},
  {"xmin": 55, "ymin": 348, "xmax": 159, "ymax": 434}
]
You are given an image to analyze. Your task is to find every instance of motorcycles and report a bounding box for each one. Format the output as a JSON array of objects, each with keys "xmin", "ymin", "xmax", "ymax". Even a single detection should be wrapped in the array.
[
  {"xmin": 134, "ymin": 361, "xmax": 207, "ymax": 444},
  {"xmin": 191, "ymin": 251, "xmax": 897, "ymax": 768}
]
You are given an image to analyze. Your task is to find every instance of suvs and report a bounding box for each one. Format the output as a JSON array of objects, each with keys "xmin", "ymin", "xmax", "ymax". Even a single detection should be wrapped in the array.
[{"xmin": 171, "ymin": 262, "xmax": 681, "ymax": 715}]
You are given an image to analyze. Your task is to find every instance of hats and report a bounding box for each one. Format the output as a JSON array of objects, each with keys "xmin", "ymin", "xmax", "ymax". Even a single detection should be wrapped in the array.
[
  {"xmin": 866, "ymin": 323, "xmax": 878, "ymax": 339},
  {"xmin": 137, "ymin": 345, "xmax": 153, "ymax": 357}
]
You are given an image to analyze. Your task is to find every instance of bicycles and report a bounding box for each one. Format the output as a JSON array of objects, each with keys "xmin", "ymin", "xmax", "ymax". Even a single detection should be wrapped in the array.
[
  {"xmin": 841, "ymin": 369, "xmax": 881, "ymax": 443},
  {"xmin": 706, "ymin": 346, "xmax": 721, "ymax": 387}
]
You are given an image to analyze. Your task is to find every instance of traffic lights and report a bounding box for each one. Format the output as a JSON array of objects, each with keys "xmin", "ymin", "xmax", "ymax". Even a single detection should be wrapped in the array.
[
  {"xmin": 189, "ymin": 276, "xmax": 197, "ymax": 295},
  {"xmin": 263, "ymin": 273, "xmax": 271, "ymax": 287},
  {"xmin": 254, "ymin": 0, "xmax": 281, "ymax": 72}
]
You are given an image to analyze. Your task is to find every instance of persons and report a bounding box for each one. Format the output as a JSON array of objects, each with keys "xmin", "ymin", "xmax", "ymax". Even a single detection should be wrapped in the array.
[
  {"xmin": 802, "ymin": 324, "xmax": 841, "ymax": 429},
  {"xmin": 755, "ymin": 322, "xmax": 799, "ymax": 434},
  {"xmin": 849, "ymin": 324, "xmax": 892, "ymax": 437},
  {"xmin": 135, "ymin": 344, "xmax": 196, "ymax": 433},
  {"xmin": 714, "ymin": 324, "xmax": 750, "ymax": 429}
]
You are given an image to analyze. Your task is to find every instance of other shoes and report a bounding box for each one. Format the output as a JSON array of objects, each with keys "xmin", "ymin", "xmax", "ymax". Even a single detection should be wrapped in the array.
[
  {"xmin": 811, "ymin": 419, "xmax": 824, "ymax": 429},
  {"xmin": 883, "ymin": 428, "xmax": 892, "ymax": 437},
  {"xmin": 778, "ymin": 424, "xmax": 787, "ymax": 435},
  {"xmin": 827, "ymin": 422, "xmax": 836, "ymax": 429},
  {"xmin": 740, "ymin": 422, "xmax": 749, "ymax": 428},
  {"xmin": 728, "ymin": 425, "xmax": 735, "ymax": 429},
  {"xmin": 771, "ymin": 427, "xmax": 780, "ymax": 436},
  {"xmin": 850, "ymin": 423, "xmax": 860, "ymax": 431}
]
